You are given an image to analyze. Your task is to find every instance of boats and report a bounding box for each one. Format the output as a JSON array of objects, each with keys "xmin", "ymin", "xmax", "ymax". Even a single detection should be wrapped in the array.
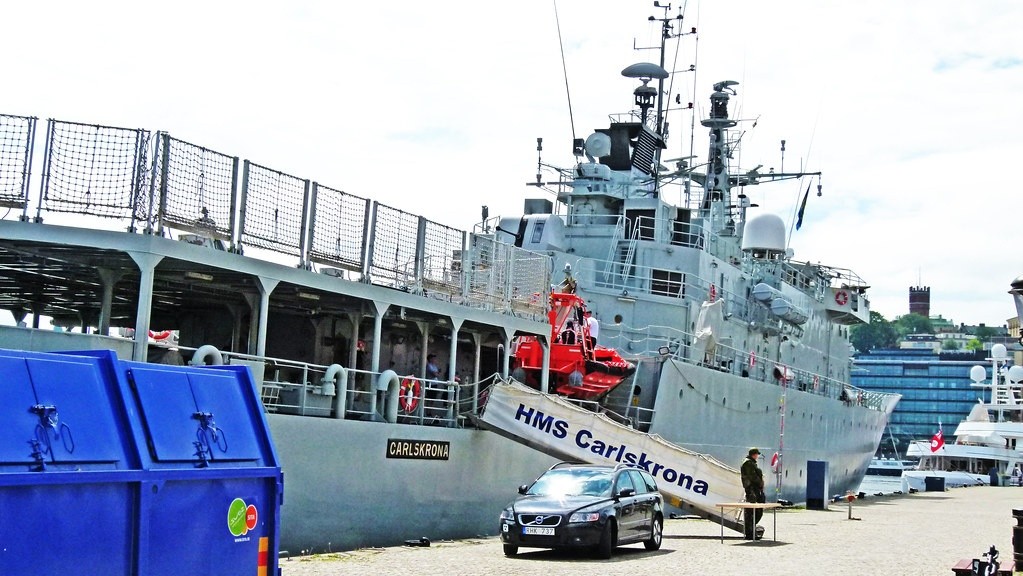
[
  {"xmin": 864, "ymin": 457, "xmax": 918, "ymax": 474},
  {"xmin": 903, "ymin": 342, "xmax": 1023, "ymax": 493},
  {"xmin": 0, "ymin": 0, "xmax": 903, "ymax": 557}
]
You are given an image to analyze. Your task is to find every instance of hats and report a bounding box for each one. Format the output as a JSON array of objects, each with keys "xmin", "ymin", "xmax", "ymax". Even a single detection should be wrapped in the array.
[
  {"xmin": 749, "ymin": 449, "xmax": 761, "ymax": 455},
  {"xmin": 427, "ymin": 354, "xmax": 437, "ymax": 361}
]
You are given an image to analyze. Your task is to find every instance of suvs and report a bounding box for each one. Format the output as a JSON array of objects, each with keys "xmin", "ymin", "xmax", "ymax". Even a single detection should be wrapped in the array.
[{"xmin": 500, "ymin": 463, "xmax": 664, "ymax": 560}]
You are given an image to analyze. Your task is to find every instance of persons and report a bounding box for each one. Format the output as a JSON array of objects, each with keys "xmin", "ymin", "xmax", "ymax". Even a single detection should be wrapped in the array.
[
  {"xmin": 583, "ymin": 311, "xmax": 598, "ymax": 355},
  {"xmin": 563, "ymin": 263, "xmax": 572, "ymax": 280},
  {"xmin": 426, "ymin": 354, "xmax": 440, "ymax": 410},
  {"xmin": 987, "ymin": 544, "xmax": 1000, "ymax": 571},
  {"xmin": 741, "ymin": 448, "xmax": 765, "ymax": 540},
  {"xmin": 562, "ymin": 321, "xmax": 577, "ymax": 344},
  {"xmin": 454, "ymin": 372, "xmax": 462, "ymax": 382},
  {"xmin": 576, "ymin": 162, "xmax": 584, "ymax": 175}
]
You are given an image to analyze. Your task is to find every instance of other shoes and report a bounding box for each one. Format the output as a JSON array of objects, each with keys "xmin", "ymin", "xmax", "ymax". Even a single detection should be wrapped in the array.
[{"xmin": 746, "ymin": 533, "xmax": 762, "ymax": 539}]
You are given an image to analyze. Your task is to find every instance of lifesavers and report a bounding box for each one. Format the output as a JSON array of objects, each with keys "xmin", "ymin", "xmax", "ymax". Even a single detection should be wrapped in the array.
[
  {"xmin": 748, "ymin": 350, "xmax": 755, "ymax": 368},
  {"xmin": 709, "ymin": 283, "xmax": 716, "ymax": 301},
  {"xmin": 399, "ymin": 375, "xmax": 421, "ymax": 412},
  {"xmin": 835, "ymin": 291, "xmax": 848, "ymax": 305},
  {"xmin": 813, "ymin": 376, "xmax": 819, "ymax": 389}
]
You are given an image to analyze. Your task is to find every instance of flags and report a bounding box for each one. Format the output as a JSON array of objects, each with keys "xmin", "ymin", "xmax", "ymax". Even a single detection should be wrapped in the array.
[
  {"xmin": 795, "ymin": 185, "xmax": 811, "ymax": 231},
  {"xmin": 930, "ymin": 430, "xmax": 945, "ymax": 452}
]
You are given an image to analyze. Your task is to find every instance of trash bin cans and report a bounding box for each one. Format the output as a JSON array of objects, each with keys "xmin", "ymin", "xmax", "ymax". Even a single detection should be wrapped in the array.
[
  {"xmin": 0, "ymin": 348, "xmax": 286, "ymax": 576},
  {"xmin": 1012, "ymin": 508, "xmax": 1023, "ymax": 572}
]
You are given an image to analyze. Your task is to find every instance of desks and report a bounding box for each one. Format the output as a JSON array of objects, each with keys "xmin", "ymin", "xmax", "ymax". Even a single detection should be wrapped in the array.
[{"xmin": 717, "ymin": 503, "xmax": 782, "ymax": 544}]
